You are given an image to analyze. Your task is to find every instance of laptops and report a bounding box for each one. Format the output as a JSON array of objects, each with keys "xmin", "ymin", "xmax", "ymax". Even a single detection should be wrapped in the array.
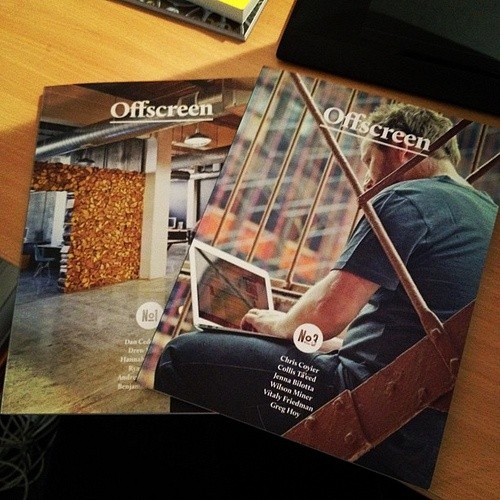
[{"xmin": 188, "ymin": 239, "xmax": 288, "ymax": 339}]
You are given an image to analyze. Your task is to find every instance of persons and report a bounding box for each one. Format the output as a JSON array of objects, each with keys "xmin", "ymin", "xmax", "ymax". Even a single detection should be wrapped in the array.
[{"xmin": 154, "ymin": 102, "xmax": 500, "ymax": 490}]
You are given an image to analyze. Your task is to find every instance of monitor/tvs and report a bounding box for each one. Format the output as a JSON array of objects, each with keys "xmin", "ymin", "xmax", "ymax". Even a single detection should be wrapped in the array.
[{"xmin": 169, "ymin": 218, "xmax": 176, "ymax": 229}]
